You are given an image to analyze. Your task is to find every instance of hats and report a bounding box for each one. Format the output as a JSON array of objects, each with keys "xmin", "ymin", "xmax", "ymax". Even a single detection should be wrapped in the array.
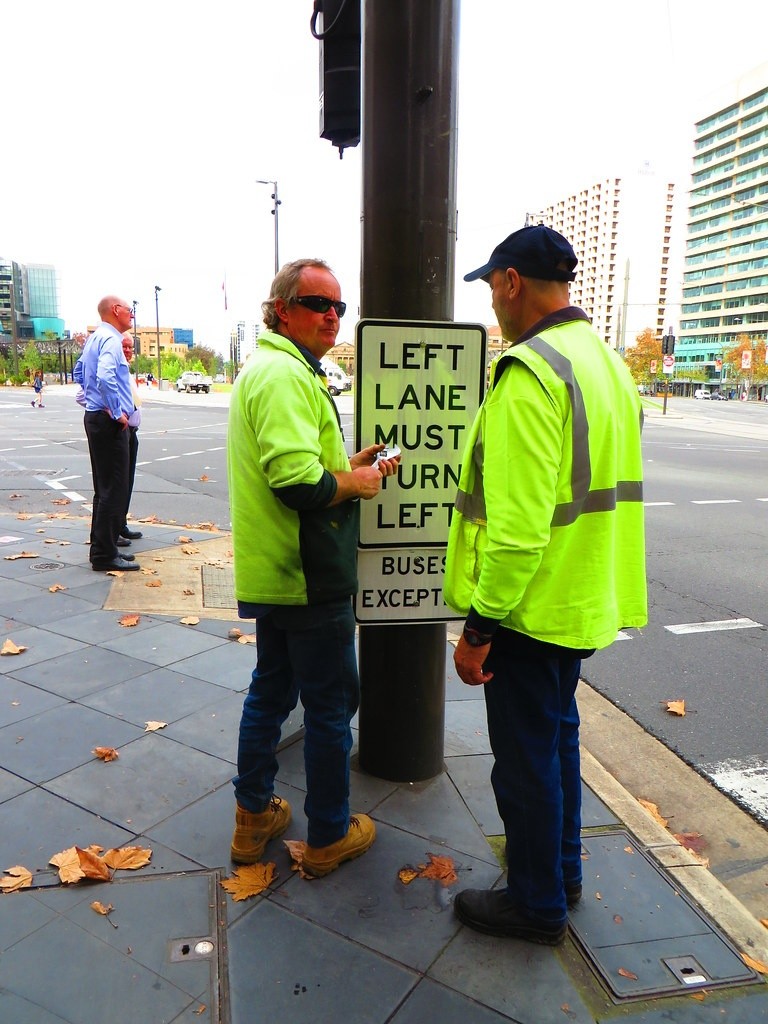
[{"xmin": 463, "ymin": 224, "xmax": 578, "ymax": 282}]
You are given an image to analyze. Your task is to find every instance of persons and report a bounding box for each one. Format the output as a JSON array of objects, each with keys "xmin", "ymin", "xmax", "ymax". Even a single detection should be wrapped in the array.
[
  {"xmin": 148, "ymin": 372, "xmax": 153, "ymax": 381},
  {"xmin": 73, "ymin": 295, "xmax": 142, "ymax": 571},
  {"xmin": 227, "ymin": 258, "xmax": 401, "ymax": 876},
  {"xmin": 443, "ymin": 223, "xmax": 648, "ymax": 946},
  {"xmin": 31, "ymin": 372, "xmax": 45, "ymax": 408}
]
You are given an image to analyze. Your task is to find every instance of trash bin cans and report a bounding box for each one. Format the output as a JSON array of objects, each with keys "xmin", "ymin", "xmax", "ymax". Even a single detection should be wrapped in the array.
[{"xmin": 161, "ymin": 379, "xmax": 169, "ymax": 390}]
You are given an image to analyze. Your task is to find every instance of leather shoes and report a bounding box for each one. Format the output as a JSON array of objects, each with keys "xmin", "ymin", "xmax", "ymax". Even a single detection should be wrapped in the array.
[
  {"xmin": 564, "ymin": 882, "xmax": 583, "ymax": 900},
  {"xmin": 90, "ymin": 552, "xmax": 140, "ymax": 571},
  {"xmin": 454, "ymin": 886, "xmax": 567, "ymax": 945}
]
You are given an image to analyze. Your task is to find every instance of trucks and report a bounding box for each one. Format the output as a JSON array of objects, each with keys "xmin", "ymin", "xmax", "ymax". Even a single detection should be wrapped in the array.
[
  {"xmin": 637, "ymin": 385, "xmax": 653, "ymax": 396},
  {"xmin": 319, "ymin": 357, "xmax": 352, "ymax": 395},
  {"xmin": 176, "ymin": 371, "xmax": 213, "ymax": 393}
]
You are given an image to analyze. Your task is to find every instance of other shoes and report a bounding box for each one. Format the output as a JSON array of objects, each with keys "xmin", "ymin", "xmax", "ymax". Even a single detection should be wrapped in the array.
[
  {"xmin": 123, "ymin": 531, "xmax": 142, "ymax": 539},
  {"xmin": 31, "ymin": 401, "xmax": 35, "ymax": 407},
  {"xmin": 38, "ymin": 404, "xmax": 45, "ymax": 407},
  {"xmin": 116, "ymin": 535, "xmax": 131, "ymax": 546}
]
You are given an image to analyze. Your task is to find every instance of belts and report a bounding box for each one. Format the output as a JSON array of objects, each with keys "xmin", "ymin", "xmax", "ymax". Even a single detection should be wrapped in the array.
[{"xmin": 129, "ymin": 425, "xmax": 138, "ymax": 433}]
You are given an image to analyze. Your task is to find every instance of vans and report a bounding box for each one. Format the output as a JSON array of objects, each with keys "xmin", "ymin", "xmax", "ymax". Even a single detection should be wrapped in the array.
[{"xmin": 695, "ymin": 390, "xmax": 712, "ymax": 399}]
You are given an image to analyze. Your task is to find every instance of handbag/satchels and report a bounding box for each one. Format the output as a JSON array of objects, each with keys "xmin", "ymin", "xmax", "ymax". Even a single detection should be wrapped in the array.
[{"xmin": 32, "ymin": 378, "xmax": 39, "ymax": 388}]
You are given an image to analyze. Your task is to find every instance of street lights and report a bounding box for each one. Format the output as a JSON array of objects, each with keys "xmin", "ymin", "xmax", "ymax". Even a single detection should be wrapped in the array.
[
  {"xmin": 699, "ymin": 338, "xmax": 723, "ymax": 393},
  {"xmin": 132, "ymin": 300, "xmax": 139, "ymax": 388},
  {"xmin": 154, "ymin": 286, "xmax": 165, "ymax": 387},
  {"xmin": 257, "ymin": 180, "xmax": 282, "ymax": 276}
]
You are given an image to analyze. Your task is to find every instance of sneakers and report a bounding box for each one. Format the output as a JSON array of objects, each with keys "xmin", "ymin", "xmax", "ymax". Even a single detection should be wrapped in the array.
[
  {"xmin": 231, "ymin": 793, "xmax": 291, "ymax": 864},
  {"xmin": 301, "ymin": 814, "xmax": 375, "ymax": 877}
]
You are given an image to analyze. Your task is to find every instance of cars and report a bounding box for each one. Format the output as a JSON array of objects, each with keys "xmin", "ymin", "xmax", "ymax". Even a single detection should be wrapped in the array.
[
  {"xmin": 711, "ymin": 392, "xmax": 729, "ymax": 401},
  {"xmin": 134, "ymin": 374, "xmax": 149, "ymax": 382}
]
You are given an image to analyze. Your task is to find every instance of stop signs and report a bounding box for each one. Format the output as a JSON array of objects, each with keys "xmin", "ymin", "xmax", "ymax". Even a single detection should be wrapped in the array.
[{"xmin": 664, "ymin": 356, "xmax": 674, "ymax": 367}]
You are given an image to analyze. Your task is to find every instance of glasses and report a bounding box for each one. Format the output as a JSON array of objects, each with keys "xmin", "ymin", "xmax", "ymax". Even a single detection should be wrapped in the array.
[
  {"xmin": 117, "ymin": 305, "xmax": 133, "ymax": 313},
  {"xmin": 123, "ymin": 346, "xmax": 135, "ymax": 351},
  {"xmin": 290, "ymin": 295, "xmax": 346, "ymax": 317}
]
